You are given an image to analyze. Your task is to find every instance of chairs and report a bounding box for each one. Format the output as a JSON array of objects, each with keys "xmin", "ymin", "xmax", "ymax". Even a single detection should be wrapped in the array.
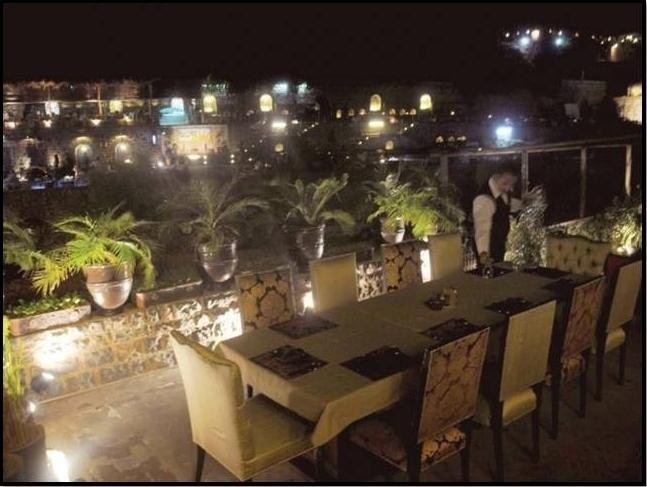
[
  {"xmin": 545, "ymin": 230, "xmax": 612, "ymax": 277},
  {"xmin": 594, "ymin": 260, "xmax": 642, "ymax": 402},
  {"xmin": 428, "ymin": 232, "xmax": 464, "ymax": 279},
  {"xmin": 308, "ymin": 252, "xmax": 358, "ymax": 312},
  {"xmin": 548, "ymin": 271, "xmax": 607, "ymax": 440},
  {"xmin": 338, "ymin": 323, "xmax": 493, "ymax": 483},
  {"xmin": 234, "ymin": 264, "xmax": 296, "ymax": 333},
  {"xmin": 380, "ymin": 239, "xmax": 423, "ymax": 295},
  {"xmin": 168, "ymin": 328, "xmax": 326, "ymax": 482},
  {"xmin": 472, "ymin": 299, "xmax": 558, "ymax": 482}
]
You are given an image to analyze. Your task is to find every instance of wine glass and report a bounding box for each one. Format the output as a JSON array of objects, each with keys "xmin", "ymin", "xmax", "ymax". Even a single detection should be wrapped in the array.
[{"xmin": 476, "ymin": 265, "xmax": 493, "ymax": 304}]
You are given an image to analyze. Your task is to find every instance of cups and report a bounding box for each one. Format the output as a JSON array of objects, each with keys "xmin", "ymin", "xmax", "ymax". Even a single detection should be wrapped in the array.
[
  {"xmin": 443, "ymin": 287, "xmax": 458, "ymax": 307},
  {"xmin": 423, "ymin": 299, "xmax": 446, "ymax": 312},
  {"xmin": 274, "ymin": 344, "xmax": 295, "ymax": 358}
]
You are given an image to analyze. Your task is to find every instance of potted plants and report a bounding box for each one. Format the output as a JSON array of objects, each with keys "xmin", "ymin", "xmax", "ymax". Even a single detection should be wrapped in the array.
[
  {"xmin": 264, "ymin": 174, "xmax": 355, "ymax": 266},
  {"xmin": 32, "ymin": 200, "xmax": 157, "ymax": 317},
  {"xmin": 158, "ymin": 173, "xmax": 269, "ymax": 284},
  {"xmin": 363, "ymin": 164, "xmax": 465, "ymax": 244},
  {"xmin": 3, "ymin": 315, "xmax": 45, "ymax": 465}
]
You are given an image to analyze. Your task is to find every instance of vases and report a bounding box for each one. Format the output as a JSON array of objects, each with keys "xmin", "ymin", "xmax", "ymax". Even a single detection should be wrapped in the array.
[{"xmin": 4, "ymin": 453, "xmax": 27, "ymax": 482}]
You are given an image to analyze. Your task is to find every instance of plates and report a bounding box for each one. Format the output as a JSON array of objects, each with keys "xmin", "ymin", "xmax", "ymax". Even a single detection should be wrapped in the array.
[{"xmin": 274, "ymin": 354, "xmax": 301, "ymax": 364}]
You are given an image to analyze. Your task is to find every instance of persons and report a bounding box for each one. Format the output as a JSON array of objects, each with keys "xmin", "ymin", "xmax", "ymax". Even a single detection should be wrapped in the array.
[
  {"xmin": 43, "ymin": 148, "xmax": 90, "ymax": 177},
  {"xmin": 461, "ymin": 159, "xmax": 518, "ymax": 278}
]
